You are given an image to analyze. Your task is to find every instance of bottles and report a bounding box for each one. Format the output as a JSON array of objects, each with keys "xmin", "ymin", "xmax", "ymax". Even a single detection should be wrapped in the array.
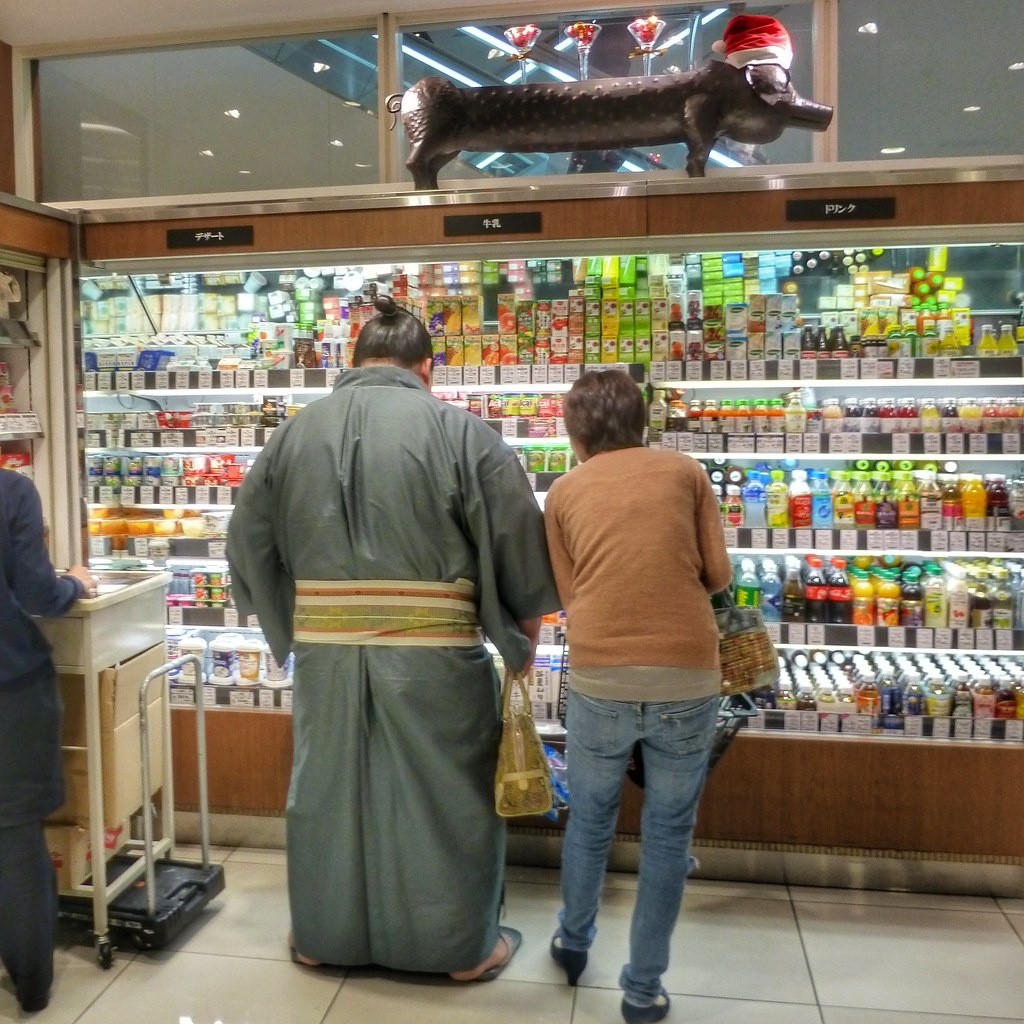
[
  {"xmin": 1005, "ymin": 290, "xmax": 1024, "ymax": 301},
  {"xmin": 696, "ymin": 459, "xmax": 1024, "ymax": 530},
  {"xmin": 802, "ymin": 302, "xmax": 1024, "ymax": 359},
  {"xmin": 296, "ymin": 277, "xmax": 323, "ymax": 292},
  {"xmin": 792, "ymin": 249, "xmax": 885, "ymax": 275},
  {"xmin": 753, "ymin": 653, "xmax": 1024, "ymax": 742},
  {"xmin": 905, "ymin": 266, "xmax": 944, "ymax": 306},
  {"xmin": 727, "ymin": 555, "xmax": 1024, "ymax": 628},
  {"xmin": 650, "ymin": 389, "xmax": 1024, "ymax": 434},
  {"xmin": 783, "ymin": 281, "xmax": 798, "ymax": 295}
]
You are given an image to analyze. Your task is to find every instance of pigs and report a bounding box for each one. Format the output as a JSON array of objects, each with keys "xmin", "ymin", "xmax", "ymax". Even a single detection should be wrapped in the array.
[{"xmin": 383, "ymin": 59, "xmax": 835, "ymax": 191}]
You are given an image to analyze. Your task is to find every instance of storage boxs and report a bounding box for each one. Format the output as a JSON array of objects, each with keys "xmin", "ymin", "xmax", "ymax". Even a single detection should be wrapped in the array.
[
  {"xmin": 43, "ymin": 816, "xmax": 132, "ymax": 890},
  {"xmin": 41, "ymin": 641, "xmax": 167, "ymax": 827}
]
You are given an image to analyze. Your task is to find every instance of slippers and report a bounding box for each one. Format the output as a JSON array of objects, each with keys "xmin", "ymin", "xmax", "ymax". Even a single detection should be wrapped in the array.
[{"xmin": 475, "ymin": 925, "xmax": 523, "ymax": 982}]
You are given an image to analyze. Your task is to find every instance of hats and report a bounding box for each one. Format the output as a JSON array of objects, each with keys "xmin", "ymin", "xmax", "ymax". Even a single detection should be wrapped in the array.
[{"xmin": 711, "ymin": 14, "xmax": 793, "ymax": 73}]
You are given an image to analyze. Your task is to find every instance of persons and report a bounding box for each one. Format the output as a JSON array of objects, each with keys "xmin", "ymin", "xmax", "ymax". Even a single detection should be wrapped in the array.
[
  {"xmin": 546, "ymin": 370, "xmax": 732, "ymax": 1024},
  {"xmin": 0, "ymin": 466, "xmax": 99, "ymax": 1011},
  {"xmin": 226, "ymin": 296, "xmax": 564, "ymax": 982}
]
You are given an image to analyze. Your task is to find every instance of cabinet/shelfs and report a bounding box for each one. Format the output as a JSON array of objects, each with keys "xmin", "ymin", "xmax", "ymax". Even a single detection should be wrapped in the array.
[
  {"xmin": 0, "ymin": 193, "xmax": 83, "ymax": 570},
  {"xmin": 78, "ymin": 162, "xmax": 1024, "ymax": 899},
  {"xmin": 50, "ymin": 567, "xmax": 174, "ymax": 971}
]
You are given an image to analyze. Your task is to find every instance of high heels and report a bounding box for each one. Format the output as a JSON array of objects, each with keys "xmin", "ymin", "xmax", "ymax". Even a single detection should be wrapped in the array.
[
  {"xmin": 622, "ymin": 986, "xmax": 670, "ymax": 1024},
  {"xmin": 551, "ymin": 927, "xmax": 588, "ymax": 986}
]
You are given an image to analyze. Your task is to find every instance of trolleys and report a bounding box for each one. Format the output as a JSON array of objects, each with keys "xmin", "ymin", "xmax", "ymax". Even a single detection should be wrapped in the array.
[
  {"xmin": 55, "ymin": 653, "xmax": 229, "ymax": 954},
  {"xmin": 26, "ymin": 560, "xmax": 177, "ymax": 971}
]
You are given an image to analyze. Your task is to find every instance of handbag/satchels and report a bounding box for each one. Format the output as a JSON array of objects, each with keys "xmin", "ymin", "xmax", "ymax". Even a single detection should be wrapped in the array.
[
  {"xmin": 710, "ymin": 587, "xmax": 780, "ymax": 696},
  {"xmin": 494, "ymin": 670, "xmax": 553, "ymax": 816}
]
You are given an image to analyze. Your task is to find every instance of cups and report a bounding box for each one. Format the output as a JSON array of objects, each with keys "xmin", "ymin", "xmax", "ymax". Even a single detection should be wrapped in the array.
[
  {"xmin": 81, "ymin": 279, "xmax": 103, "ymax": 302},
  {"xmin": 167, "ymin": 635, "xmax": 288, "ymax": 680},
  {"xmin": 244, "ymin": 272, "xmax": 267, "ymax": 294}
]
